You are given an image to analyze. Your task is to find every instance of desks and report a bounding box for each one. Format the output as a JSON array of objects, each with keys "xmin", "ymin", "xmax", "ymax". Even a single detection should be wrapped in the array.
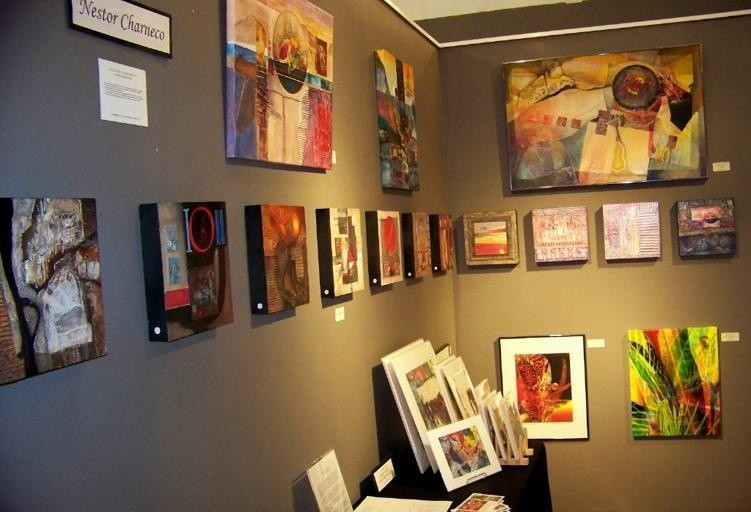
[{"xmin": 353, "ymin": 440, "xmax": 550, "ymax": 512}]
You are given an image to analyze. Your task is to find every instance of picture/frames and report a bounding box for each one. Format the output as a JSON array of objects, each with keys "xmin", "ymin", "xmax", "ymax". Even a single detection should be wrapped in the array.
[
  {"xmin": 461, "ymin": 210, "xmax": 520, "ymax": 266},
  {"xmin": 379, "ymin": 334, "xmax": 590, "ymax": 492},
  {"xmin": 501, "ymin": 43, "xmax": 711, "ymax": 192}
]
